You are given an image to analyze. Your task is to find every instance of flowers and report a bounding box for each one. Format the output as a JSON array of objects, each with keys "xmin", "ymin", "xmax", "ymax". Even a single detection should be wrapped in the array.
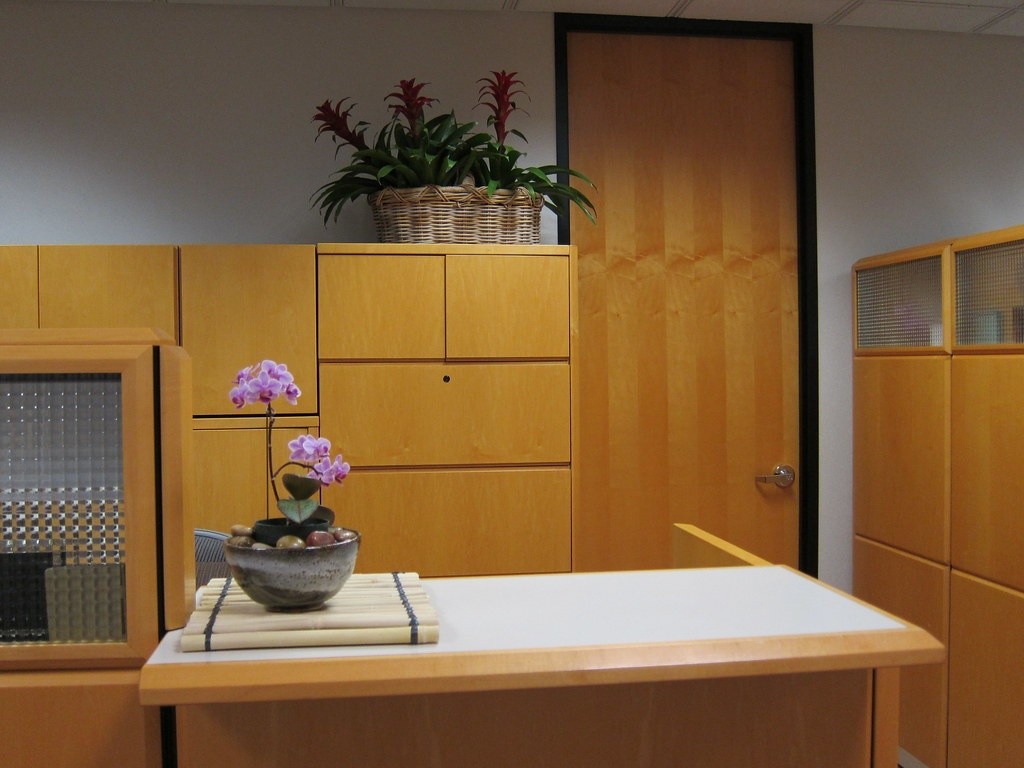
[{"xmin": 229, "ymin": 362, "xmax": 350, "ymax": 525}]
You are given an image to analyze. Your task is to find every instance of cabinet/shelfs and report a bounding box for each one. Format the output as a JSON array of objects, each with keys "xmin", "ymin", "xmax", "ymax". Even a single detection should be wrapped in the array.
[
  {"xmin": 0, "ymin": 242, "xmax": 319, "ymax": 416},
  {"xmin": 852, "ymin": 224, "xmax": 1024, "ymax": 768},
  {"xmin": 0, "ymin": 345, "xmax": 193, "ymax": 768},
  {"xmin": 191, "ymin": 416, "xmax": 317, "ymax": 536},
  {"xmin": 318, "ymin": 242, "xmax": 578, "ymax": 579}
]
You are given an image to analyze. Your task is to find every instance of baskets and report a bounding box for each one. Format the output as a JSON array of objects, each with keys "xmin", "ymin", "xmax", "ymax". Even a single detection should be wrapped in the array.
[{"xmin": 368, "ymin": 186, "xmax": 545, "ymax": 244}]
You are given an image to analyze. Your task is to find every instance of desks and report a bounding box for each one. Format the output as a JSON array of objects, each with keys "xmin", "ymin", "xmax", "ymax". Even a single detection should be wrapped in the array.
[{"xmin": 138, "ymin": 563, "xmax": 946, "ymax": 768}]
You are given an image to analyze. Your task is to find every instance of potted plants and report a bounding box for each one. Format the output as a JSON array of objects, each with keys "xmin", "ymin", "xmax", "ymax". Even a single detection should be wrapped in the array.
[{"xmin": 310, "ymin": 69, "xmax": 598, "ymax": 244}]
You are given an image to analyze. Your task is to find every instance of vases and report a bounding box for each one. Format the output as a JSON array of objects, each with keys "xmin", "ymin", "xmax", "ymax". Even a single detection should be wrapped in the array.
[
  {"xmin": 254, "ymin": 518, "xmax": 329, "ymax": 547},
  {"xmin": 224, "ymin": 529, "xmax": 362, "ymax": 612}
]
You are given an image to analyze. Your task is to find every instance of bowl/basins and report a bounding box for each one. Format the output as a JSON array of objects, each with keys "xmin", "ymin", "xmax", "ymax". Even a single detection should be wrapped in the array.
[{"xmin": 225, "ymin": 526, "xmax": 360, "ymax": 613}]
